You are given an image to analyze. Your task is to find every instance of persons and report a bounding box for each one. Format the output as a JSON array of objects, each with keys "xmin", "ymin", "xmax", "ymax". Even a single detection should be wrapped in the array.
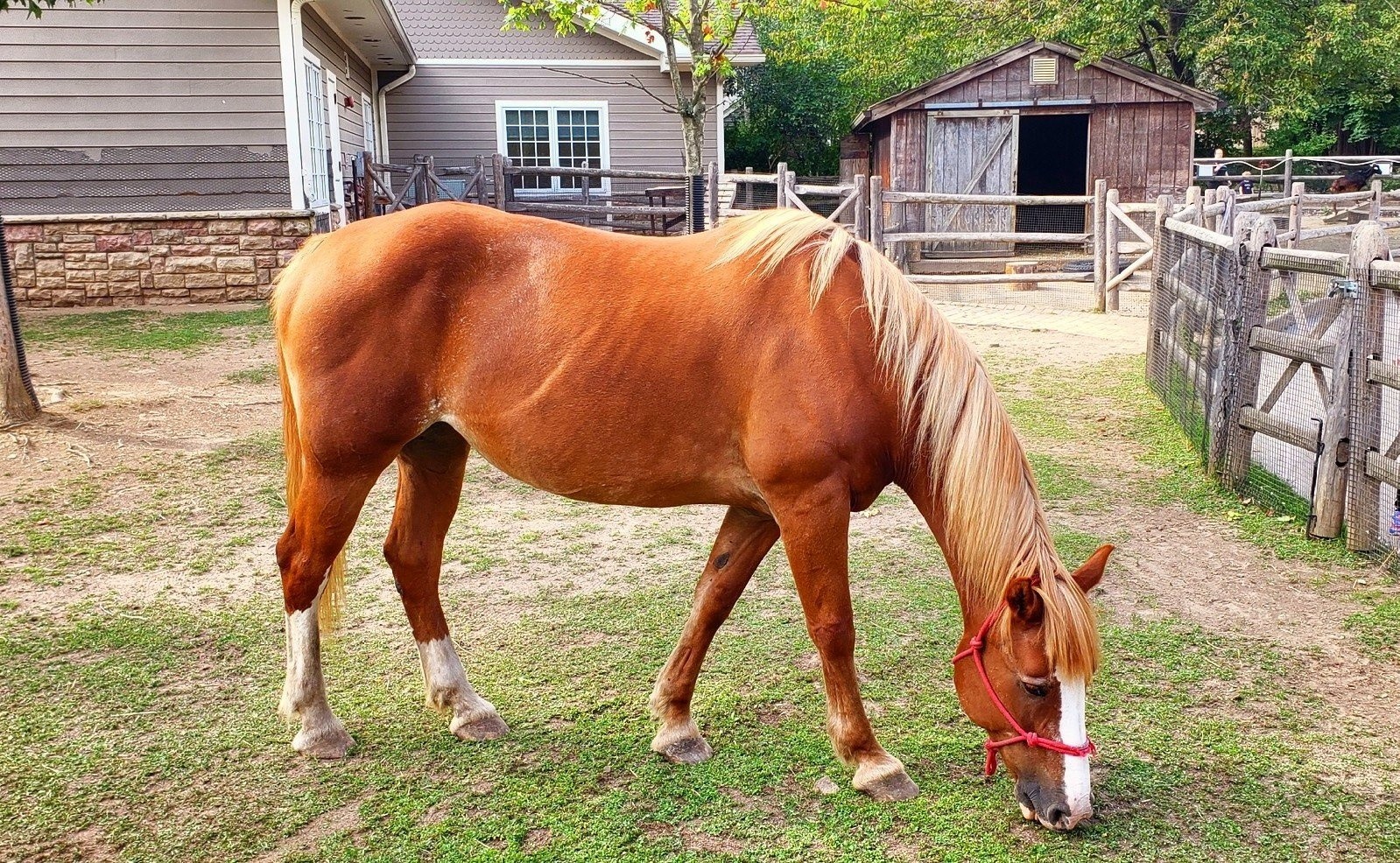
[
  {"xmin": 1239, "ymin": 171, "xmax": 1254, "ymax": 195},
  {"xmin": 1207, "ymin": 149, "xmax": 1232, "ymax": 189}
]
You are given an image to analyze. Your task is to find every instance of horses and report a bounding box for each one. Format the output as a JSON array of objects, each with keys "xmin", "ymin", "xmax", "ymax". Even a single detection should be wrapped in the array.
[{"xmin": 259, "ymin": 204, "xmax": 1117, "ymax": 836}]
